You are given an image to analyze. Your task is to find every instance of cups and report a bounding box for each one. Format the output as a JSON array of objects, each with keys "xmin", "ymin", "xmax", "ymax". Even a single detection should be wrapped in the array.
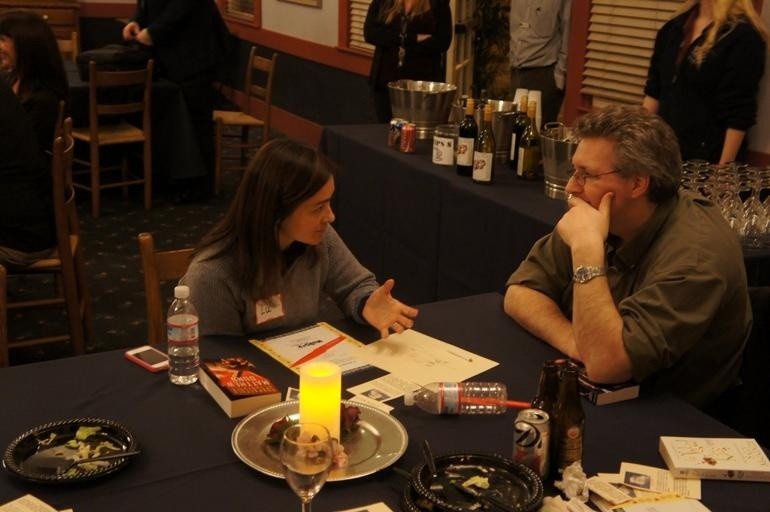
[{"xmin": 513, "ymin": 89, "xmax": 543, "ymax": 133}]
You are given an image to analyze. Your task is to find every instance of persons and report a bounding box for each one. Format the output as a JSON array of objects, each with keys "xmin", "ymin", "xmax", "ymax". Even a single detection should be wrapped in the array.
[
  {"xmin": 118, "ymin": 1, "xmax": 239, "ymax": 205},
  {"xmin": 502, "ymin": 105, "xmax": 753, "ymax": 431},
  {"xmin": 2, "ymin": 8, "xmax": 70, "ymax": 252},
  {"xmin": 361, "ymin": 1, "xmax": 454, "ymax": 123},
  {"xmin": 179, "ymin": 130, "xmax": 420, "ymax": 342},
  {"xmin": 509, "ymin": 1, "xmax": 570, "ymax": 130},
  {"xmin": 643, "ymin": 1, "xmax": 768, "ymax": 164}
]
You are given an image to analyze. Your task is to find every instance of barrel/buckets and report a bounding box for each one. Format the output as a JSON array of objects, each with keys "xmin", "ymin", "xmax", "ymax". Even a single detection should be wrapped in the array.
[
  {"xmin": 387, "ymin": 78, "xmax": 458, "ymax": 140},
  {"xmin": 538, "ymin": 121, "xmax": 581, "ymax": 203},
  {"xmin": 450, "ymin": 98, "xmax": 516, "ymax": 165}
]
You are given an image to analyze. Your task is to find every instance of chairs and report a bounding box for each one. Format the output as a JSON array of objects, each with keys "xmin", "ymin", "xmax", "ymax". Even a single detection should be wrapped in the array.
[
  {"xmin": 136, "ymin": 230, "xmax": 201, "ymax": 346},
  {"xmin": 63, "ymin": 56, "xmax": 156, "ymax": 217},
  {"xmin": 5, "ymin": 5, "xmax": 77, "ymax": 60},
  {"xmin": 0, "ymin": 116, "xmax": 90, "ymax": 368},
  {"xmin": 211, "ymin": 45, "xmax": 278, "ymax": 197}
]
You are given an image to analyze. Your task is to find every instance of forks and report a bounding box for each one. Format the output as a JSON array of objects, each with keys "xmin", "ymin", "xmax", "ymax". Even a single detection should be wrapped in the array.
[{"xmin": 420, "ymin": 438, "xmax": 449, "ymax": 503}]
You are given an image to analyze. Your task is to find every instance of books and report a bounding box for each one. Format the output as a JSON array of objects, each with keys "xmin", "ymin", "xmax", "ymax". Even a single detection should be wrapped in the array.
[
  {"xmin": 194, "ymin": 352, "xmax": 284, "ymax": 423},
  {"xmin": 659, "ymin": 433, "xmax": 770, "ymax": 486}
]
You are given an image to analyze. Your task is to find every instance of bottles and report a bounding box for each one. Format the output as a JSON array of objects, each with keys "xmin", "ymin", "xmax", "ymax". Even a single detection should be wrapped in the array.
[
  {"xmin": 403, "ymin": 381, "xmax": 508, "ymax": 415},
  {"xmin": 508, "ymin": 96, "xmax": 530, "ymax": 171},
  {"xmin": 532, "ymin": 359, "xmax": 585, "ymax": 489},
  {"xmin": 455, "ymin": 97, "xmax": 477, "ymax": 175},
  {"xmin": 472, "ymin": 107, "xmax": 496, "ymax": 184},
  {"xmin": 166, "ymin": 284, "xmax": 199, "ymax": 386},
  {"xmin": 516, "ymin": 101, "xmax": 540, "ymax": 183},
  {"xmin": 430, "ymin": 124, "xmax": 459, "ymax": 168}
]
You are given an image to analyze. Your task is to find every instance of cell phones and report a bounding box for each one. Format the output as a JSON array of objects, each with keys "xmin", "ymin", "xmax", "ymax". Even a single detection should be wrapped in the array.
[{"xmin": 124, "ymin": 345, "xmax": 170, "ymax": 372}]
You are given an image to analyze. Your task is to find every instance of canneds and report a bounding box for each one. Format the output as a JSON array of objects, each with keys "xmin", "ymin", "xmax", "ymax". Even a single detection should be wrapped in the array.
[
  {"xmin": 511, "ymin": 408, "xmax": 552, "ymax": 481},
  {"xmin": 387, "ymin": 118, "xmax": 417, "ymax": 154}
]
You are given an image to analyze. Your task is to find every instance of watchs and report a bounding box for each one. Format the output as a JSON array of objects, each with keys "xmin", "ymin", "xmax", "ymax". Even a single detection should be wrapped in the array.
[{"xmin": 574, "ymin": 265, "xmax": 608, "ymax": 286}]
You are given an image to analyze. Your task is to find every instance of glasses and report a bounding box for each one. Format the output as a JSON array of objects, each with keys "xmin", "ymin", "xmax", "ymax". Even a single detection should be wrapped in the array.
[{"xmin": 567, "ymin": 167, "xmax": 619, "ymax": 185}]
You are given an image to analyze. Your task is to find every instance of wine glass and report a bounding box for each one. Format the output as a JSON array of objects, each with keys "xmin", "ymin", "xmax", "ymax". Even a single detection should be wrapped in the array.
[
  {"xmin": 675, "ymin": 157, "xmax": 769, "ymax": 251},
  {"xmin": 277, "ymin": 423, "xmax": 333, "ymax": 512}
]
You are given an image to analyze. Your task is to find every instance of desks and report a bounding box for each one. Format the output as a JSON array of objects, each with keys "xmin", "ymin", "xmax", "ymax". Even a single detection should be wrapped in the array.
[
  {"xmin": 320, "ymin": 123, "xmax": 768, "ymax": 453},
  {"xmin": 0, "ymin": 291, "xmax": 769, "ymax": 511}
]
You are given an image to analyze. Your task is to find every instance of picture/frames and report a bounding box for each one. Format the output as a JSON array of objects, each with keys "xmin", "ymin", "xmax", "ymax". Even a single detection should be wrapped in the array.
[{"xmin": 278, "ymin": 0, "xmax": 322, "ymax": 9}]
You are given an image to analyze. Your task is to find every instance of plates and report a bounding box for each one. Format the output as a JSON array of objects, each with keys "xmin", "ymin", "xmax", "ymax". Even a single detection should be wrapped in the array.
[
  {"xmin": 3, "ymin": 417, "xmax": 136, "ymax": 483},
  {"xmin": 231, "ymin": 400, "xmax": 409, "ymax": 484},
  {"xmin": 410, "ymin": 451, "xmax": 543, "ymax": 509},
  {"xmin": 403, "ymin": 485, "xmax": 445, "ymax": 511}
]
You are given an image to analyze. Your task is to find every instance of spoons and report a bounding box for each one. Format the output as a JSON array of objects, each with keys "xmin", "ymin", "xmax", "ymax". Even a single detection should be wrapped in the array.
[
  {"xmin": 455, "ymin": 480, "xmax": 519, "ymax": 511},
  {"xmin": 33, "ymin": 448, "xmax": 142, "ymax": 476}
]
